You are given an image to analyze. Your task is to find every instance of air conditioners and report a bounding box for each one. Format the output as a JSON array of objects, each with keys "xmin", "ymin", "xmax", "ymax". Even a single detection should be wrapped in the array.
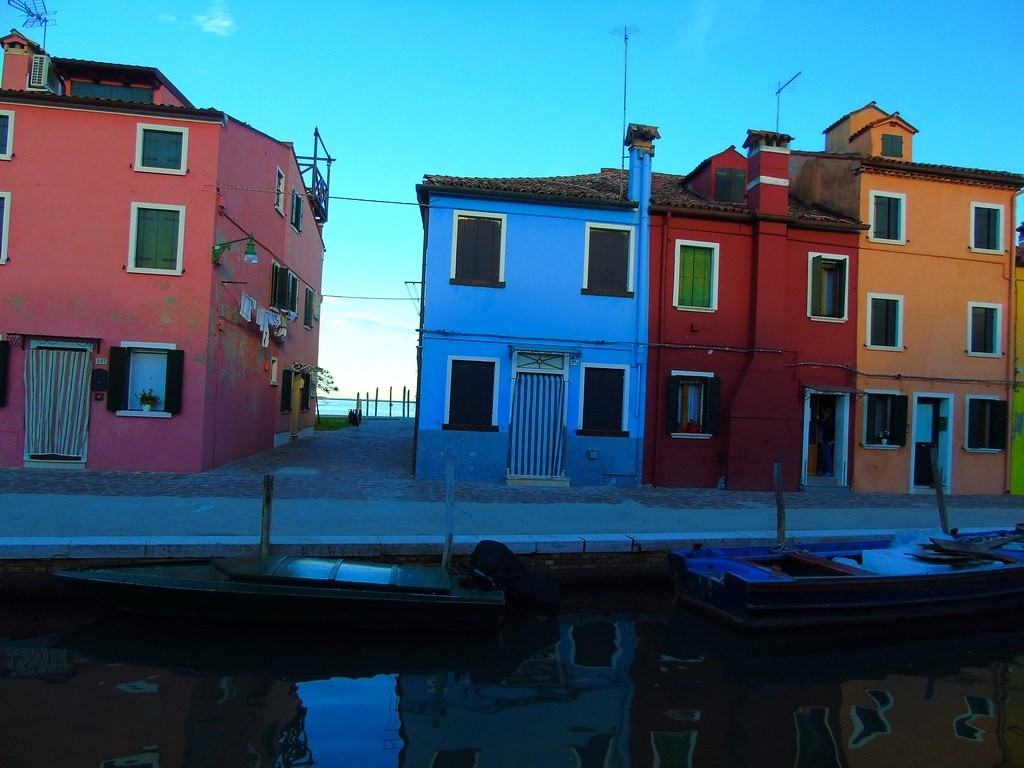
[{"xmin": 30, "ymin": 54, "xmax": 62, "ymax": 95}]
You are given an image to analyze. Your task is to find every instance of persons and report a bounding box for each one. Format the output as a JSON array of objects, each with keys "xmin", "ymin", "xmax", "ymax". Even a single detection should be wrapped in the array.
[{"xmin": 816, "ymin": 406, "xmax": 834, "ymax": 477}]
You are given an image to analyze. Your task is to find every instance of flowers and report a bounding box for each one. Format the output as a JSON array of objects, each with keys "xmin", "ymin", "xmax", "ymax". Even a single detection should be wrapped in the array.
[
  {"xmin": 880, "ymin": 430, "xmax": 890, "ymax": 440},
  {"xmin": 134, "ymin": 388, "xmax": 161, "ymax": 407}
]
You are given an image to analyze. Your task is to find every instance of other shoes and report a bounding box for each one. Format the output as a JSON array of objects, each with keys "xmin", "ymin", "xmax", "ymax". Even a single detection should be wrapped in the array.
[
  {"xmin": 824, "ymin": 473, "xmax": 832, "ymax": 478},
  {"xmin": 817, "ymin": 472, "xmax": 823, "ymax": 476}
]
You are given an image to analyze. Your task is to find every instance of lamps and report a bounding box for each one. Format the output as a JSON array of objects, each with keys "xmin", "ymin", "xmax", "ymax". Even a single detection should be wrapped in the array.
[{"xmin": 212, "ymin": 233, "xmax": 258, "ymax": 263}]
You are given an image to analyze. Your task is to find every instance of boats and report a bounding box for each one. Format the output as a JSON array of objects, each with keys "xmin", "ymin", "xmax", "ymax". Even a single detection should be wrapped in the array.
[
  {"xmin": 51, "ymin": 538, "xmax": 524, "ymax": 633},
  {"xmin": 668, "ymin": 523, "xmax": 1023, "ymax": 630}
]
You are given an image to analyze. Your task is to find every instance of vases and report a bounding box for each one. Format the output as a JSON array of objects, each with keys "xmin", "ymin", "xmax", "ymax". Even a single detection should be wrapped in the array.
[
  {"xmin": 142, "ymin": 404, "xmax": 151, "ymax": 411},
  {"xmin": 881, "ymin": 439, "xmax": 888, "ymax": 445}
]
